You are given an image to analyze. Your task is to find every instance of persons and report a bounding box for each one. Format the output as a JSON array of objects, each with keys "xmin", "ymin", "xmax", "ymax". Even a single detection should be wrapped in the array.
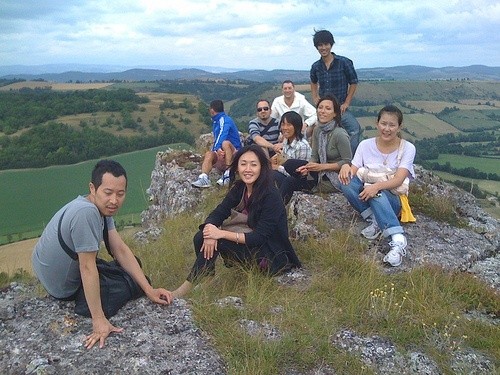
[
  {"xmin": 167, "ymin": 145, "xmax": 303, "ymax": 300},
  {"xmin": 278, "ymin": 94, "xmax": 353, "ymax": 205},
  {"xmin": 310, "ymin": 30, "xmax": 362, "ymax": 158},
  {"xmin": 270, "ymin": 80, "xmax": 318, "ymax": 139},
  {"xmin": 247, "ymin": 99, "xmax": 285, "ymax": 168},
  {"xmin": 336, "ymin": 104, "xmax": 417, "ymax": 268},
  {"xmin": 269, "ymin": 111, "xmax": 312, "ymax": 188},
  {"xmin": 31, "ymin": 160, "xmax": 174, "ymax": 351},
  {"xmin": 192, "ymin": 100, "xmax": 242, "ymax": 188}
]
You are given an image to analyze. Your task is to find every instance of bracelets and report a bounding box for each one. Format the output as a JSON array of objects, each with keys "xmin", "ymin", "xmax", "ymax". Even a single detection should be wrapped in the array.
[{"xmin": 236, "ymin": 232, "xmax": 239, "ymax": 245}]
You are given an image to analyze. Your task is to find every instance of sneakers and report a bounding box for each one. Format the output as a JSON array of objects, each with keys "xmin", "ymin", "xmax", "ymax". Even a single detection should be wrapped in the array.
[
  {"xmin": 216, "ymin": 169, "xmax": 230, "ymax": 187},
  {"xmin": 192, "ymin": 173, "xmax": 212, "ymax": 188},
  {"xmin": 382, "ymin": 235, "xmax": 407, "ymax": 267},
  {"xmin": 361, "ymin": 219, "xmax": 383, "ymax": 240}
]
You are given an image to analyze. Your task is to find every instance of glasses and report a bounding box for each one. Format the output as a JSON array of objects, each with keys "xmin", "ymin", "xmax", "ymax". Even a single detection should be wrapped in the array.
[{"xmin": 256, "ymin": 106, "xmax": 270, "ymax": 112}]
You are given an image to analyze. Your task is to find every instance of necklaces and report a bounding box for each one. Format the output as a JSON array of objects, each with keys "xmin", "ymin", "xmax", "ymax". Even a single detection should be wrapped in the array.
[{"xmin": 379, "ymin": 136, "xmax": 398, "ymax": 165}]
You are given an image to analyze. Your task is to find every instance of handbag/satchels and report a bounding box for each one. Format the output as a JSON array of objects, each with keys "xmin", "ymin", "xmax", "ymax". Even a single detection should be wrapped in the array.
[
  {"xmin": 243, "ymin": 136, "xmax": 255, "ymax": 146},
  {"xmin": 358, "ymin": 166, "xmax": 409, "ymax": 195},
  {"xmin": 74, "ymin": 254, "xmax": 151, "ymax": 320},
  {"xmin": 221, "ymin": 185, "xmax": 253, "ymax": 233}
]
